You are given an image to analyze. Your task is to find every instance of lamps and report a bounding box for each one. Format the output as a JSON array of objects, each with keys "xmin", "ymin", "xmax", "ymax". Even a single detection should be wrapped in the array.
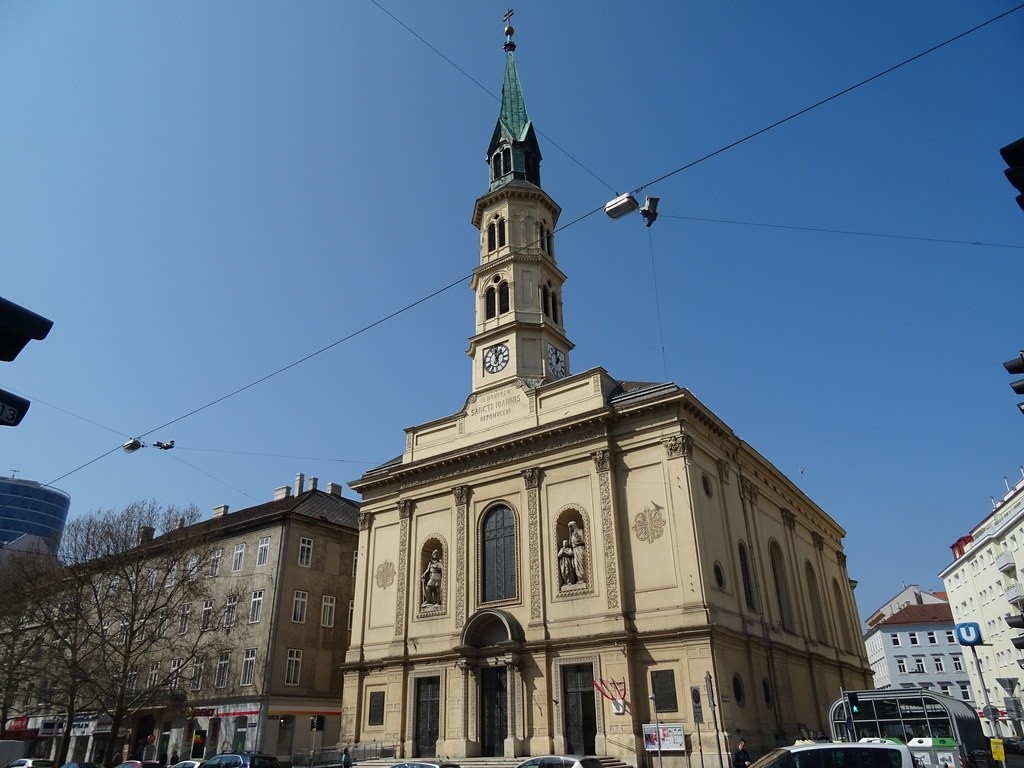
[
  {"xmin": 604, "ymin": 192, "xmax": 638, "ymax": 219},
  {"xmin": 484, "ymin": 344, "xmax": 509, "ymax": 374},
  {"xmin": 547, "ymin": 347, "xmax": 567, "ymax": 379},
  {"xmin": 122, "ymin": 439, "xmax": 141, "ymax": 454}
]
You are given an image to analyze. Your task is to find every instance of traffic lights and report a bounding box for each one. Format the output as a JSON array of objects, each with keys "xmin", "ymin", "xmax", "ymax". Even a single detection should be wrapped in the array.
[
  {"xmin": 1004, "ymin": 614, "xmax": 1024, "ymax": 670},
  {"xmin": 847, "ymin": 693, "xmax": 862, "ymax": 716}
]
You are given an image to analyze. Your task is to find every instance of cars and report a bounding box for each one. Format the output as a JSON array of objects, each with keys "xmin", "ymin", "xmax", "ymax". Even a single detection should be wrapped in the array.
[
  {"xmin": 748, "ymin": 740, "xmax": 916, "ymax": 768},
  {"xmin": 390, "ymin": 760, "xmax": 460, "ymax": 768},
  {"xmin": 170, "ymin": 760, "xmax": 201, "ymax": 768},
  {"xmin": 516, "ymin": 755, "xmax": 602, "ymax": 768},
  {"xmin": 5, "ymin": 758, "xmax": 97, "ymax": 768}
]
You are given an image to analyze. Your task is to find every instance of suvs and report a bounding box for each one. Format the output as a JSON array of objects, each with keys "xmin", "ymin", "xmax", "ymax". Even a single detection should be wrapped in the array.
[
  {"xmin": 199, "ymin": 749, "xmax": 282, "ymax": 768},
  {"xmin": 113, "ymin": 759, "xmax": 161, "ymax": 768}
]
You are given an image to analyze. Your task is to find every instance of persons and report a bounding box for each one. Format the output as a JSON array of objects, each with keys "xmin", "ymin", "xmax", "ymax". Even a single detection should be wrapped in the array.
[
  {"xmin": 801, "ymin": 728, "xmax": 830, "ymax": 743},
  {"xmin": 731, "ymin": 739, "xmax": 752, "ymax": 768},
  {"xmin": 94, "ymin": 752, "xmax": 102, "ymax": 768},
  {"xmin": 421, "ymin": 550, "xmax": 443, "ymax": 607},
  {"xmin": 170, "ymin": 752, "xmax": 179, "ymax": 765},
  {"xmin": 556, "ymin": 540, "xmax": 574, "ymax": 587},
  {"xmin": 342, "ymin": 747, "xmax": 353, "ymax": 768},
  {"xmin": 127, "ymin": 752, "xmax": 134, "ymax": 761},
  {"xmin": 159, "ymin": 751, "xmax": 167, "ymax": 768},
  {"xmin": 112, "ymin": 750, "xmax": 122, "ymax": 766},
  {"xmin": 568, "ymin": 520, "xmax": 587, "ymax": 584},
  {"xmin": 135, "ymin": 749, "xmax": 142, "ymax": 761}
]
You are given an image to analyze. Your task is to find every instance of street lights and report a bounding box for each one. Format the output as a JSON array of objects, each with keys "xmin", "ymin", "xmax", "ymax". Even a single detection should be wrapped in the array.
[{"xmin": 309, "ymin": 715, "xmax": 318, "ymax": 768}]
